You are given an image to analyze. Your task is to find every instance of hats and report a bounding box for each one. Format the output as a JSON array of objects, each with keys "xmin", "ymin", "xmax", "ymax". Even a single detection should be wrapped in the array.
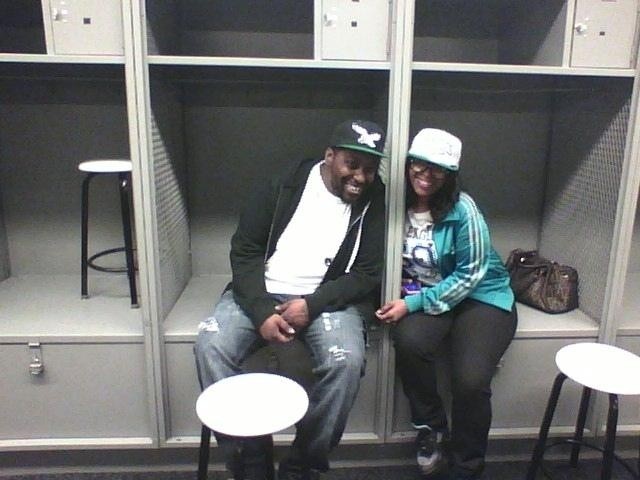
[
  {"xmin": 407, "ymin": 128, "xmax": 462, "ymax": 172},
  {"xmin": 329, "ymin": 119, "xmax": 388, "ymax": 157}
]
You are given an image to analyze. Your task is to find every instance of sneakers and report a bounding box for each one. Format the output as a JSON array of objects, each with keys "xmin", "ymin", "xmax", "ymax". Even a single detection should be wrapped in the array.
[{"xmin": 410, "ymin": 423, "xmax": 448, "ymax": 475}]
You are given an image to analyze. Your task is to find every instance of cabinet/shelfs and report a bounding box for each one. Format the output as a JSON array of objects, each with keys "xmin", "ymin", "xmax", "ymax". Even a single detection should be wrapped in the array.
[
  {"xmin": 597, "ymin": 148, "xmax": 640, "ymax": 462},
  {"xmin": 385, "ymin": 0, "xmax": 640, "ymax": 470},
  {"xmin": 0, "ymin": 0, "xmax": 159, "ymax": 479},
  {"xmin": 129, "ymin": 1, "xmax": 404, "ymax": 479}
]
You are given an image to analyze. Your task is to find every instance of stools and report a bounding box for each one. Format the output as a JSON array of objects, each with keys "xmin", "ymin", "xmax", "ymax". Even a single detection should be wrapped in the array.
[
  {"xmin": 195, "ymin": 372, "xmax": 311, "ymax": 480},
  {"xmin": 78, "ymin": 158, "xmax": 139, "ymax": 308},
  {"xmin": 525, "ymin": 342, "xmax": 640, "ymax": 480}
]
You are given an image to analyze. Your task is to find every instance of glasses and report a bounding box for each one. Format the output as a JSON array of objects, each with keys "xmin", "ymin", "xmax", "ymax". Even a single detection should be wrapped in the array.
[{"xmin": 408, "ymin": 157, "xmax": 448, "ymax": 180}]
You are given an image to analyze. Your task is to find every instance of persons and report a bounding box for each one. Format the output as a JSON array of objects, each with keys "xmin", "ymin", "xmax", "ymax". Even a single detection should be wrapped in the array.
[
  {"xmin": 374, "ymin": 127, "xmax": 519, "ymax": 480},
  {"xmin": 191, "ymin": 118, "xmax": 386, "ymax": 480}
]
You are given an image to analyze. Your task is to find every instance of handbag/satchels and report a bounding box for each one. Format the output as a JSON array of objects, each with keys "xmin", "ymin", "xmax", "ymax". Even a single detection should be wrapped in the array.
[{"xmin": 505, "ymin": 248, "xmax": 578, "ymax": 315}]
